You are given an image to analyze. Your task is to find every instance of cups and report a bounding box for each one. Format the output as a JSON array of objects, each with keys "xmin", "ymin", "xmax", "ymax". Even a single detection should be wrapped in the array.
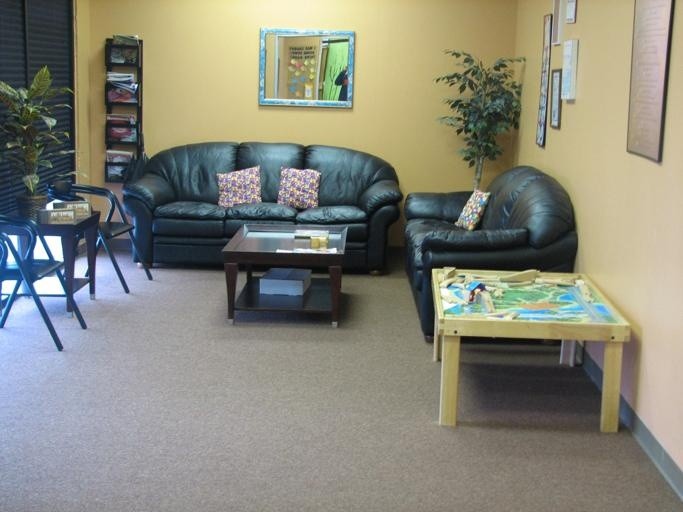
[{"xmin": 311, "ymin": 236, "xmax": 320, "ymax": 250}]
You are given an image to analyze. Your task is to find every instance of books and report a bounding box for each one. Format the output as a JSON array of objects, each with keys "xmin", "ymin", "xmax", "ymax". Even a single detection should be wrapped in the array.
[
  {"xmin": 106, "ymin": 113, "xmax": 137, "ymax": 142},
  {"xmin": 106, "ymin": 72, "xmax": 139, "ymax": 104},
  {"xmin": 106, "ymin": 150, "xmax": 133, "ymax": 179},
  {"xmin": 110, "ymin": 34, "xmax": 139, "ymax": 64}
]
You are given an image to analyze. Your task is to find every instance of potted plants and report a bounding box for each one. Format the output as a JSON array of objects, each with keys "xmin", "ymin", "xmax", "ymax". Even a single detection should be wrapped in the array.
[{"xmin": 0, "ymin": 64, "xmax": 74, "ymax": 224}]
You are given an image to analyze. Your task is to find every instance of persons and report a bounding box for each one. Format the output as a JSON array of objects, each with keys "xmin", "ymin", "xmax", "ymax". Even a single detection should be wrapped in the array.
[{"xmin": 335, "ymin": 67, "xmax": 348, "ymax": 101}]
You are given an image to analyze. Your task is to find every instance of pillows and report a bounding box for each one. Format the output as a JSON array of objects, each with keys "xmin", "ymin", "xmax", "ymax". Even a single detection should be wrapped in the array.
[
  {"xmin": 216, "ymin": 166, "xmax": 262, "ymax": 207},
  {"xmin": 276, "ymin": 167, "xmax": 320, "ymax": 209},
  {"xmin": 453, "ymin": 189, "xmax": 491, "ymax": 230}
]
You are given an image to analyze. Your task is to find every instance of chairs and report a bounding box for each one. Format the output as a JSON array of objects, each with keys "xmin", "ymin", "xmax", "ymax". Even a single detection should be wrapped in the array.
[
  {"xmin": 0, "ymin": 215, "xmax": 87, "ymax": 351},
  {"xmin": 46, "ymin": 181, "xmax": 153, "ymax": 293}
]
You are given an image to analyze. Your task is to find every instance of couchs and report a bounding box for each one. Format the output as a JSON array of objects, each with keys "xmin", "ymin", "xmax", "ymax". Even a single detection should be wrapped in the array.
[
  {"xmin": 403, "ymin": 166, "xmax": 579, "ymax": 341},
  {"xmin": 123, "ymin": 142, "xmax": 403, "ymax": 276}
]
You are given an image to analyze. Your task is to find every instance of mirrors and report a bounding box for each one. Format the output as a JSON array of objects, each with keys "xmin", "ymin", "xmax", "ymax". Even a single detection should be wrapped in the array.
[{"xmin": 259, "ymin": 29, "xmax": 354, "ymax": 108}]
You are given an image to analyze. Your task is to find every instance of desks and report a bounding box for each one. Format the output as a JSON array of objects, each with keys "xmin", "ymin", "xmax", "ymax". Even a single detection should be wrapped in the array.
[
  {"xmin": 432, "ymin": 268, "xmax": 632, "ymax": 432},
  {"xmin": 0, "ymin": 210, "xmax": 100, "ymax": 318}
]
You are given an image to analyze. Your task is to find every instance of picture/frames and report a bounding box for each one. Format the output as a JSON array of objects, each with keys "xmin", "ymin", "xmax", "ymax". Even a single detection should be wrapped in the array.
[
  {"xmin": 552, "ymin": 0, "xmax": 562, "ymax": 45},
  {"xmin": 550, "ymin": 69, "xmax": 561, "ymax": 128},
  {"xmin": 562, "ymin": 39, "xmax": 579, "ymax": 101},
  {"xmin": 536, "ymin": 14, "xmax": 552, "ymax": 147},
  {"xmin": 565, "ymin": 0, "xmax": 577, "ymax": 24}
]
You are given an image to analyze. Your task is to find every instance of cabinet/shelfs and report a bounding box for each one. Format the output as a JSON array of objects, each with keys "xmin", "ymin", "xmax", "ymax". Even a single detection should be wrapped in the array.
[{"xmin": 104, "ymin": 36, "xmax": 145, "ymax": 183}]
